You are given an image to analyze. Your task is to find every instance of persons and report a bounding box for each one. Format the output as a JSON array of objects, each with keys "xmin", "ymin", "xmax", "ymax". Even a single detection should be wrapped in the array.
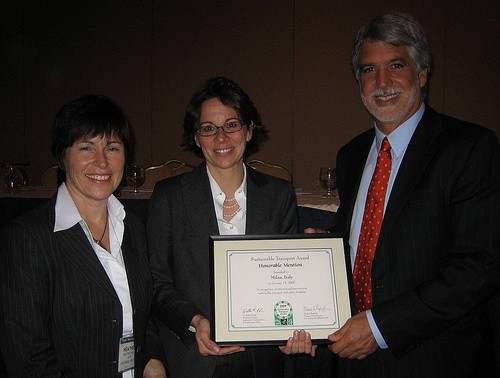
[
  {"xmin": 0, "ymin": 96, "xmax": 170, "ymax": 378},
  {"xmin": 303, "ymin": 13, "xmax": 500, "ymax": 378},
  {"xmin": 146, "ymin": 77, "xmax": 317, "ymax": 378}
]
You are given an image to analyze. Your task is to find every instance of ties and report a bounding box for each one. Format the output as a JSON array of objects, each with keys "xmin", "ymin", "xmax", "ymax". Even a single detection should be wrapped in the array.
[{"xmin": 352, "ymin": 137, "xmax": 392, "ymax": 313}]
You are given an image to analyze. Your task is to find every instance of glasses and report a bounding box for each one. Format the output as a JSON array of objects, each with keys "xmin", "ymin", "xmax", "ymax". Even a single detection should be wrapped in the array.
[{"xmin": 197, "ymin": 121, "xmax": 243, "ymax": 136}]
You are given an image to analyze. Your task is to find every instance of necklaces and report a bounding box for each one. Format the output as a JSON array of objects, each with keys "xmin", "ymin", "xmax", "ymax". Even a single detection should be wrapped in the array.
[
  {"xmin": 222, "ymin": 198, "xmax": 238, "ymax": 220},
  {"xmin": 89, "ymin": 206, "xmax": 108, "ymax": 250}
]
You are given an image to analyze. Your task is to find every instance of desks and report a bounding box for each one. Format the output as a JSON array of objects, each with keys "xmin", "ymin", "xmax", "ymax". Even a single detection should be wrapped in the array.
[{"xmin": 0, "ymin": 185, "xmax": 341, "ymax": 234}]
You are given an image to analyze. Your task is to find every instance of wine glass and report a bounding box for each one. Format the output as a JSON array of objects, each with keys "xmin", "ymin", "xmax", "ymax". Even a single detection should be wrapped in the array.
[
  {"xmin": 3, "ymin": 164, "xmax": 24, "ymax": 193},
  {"xmin": 319, "ymin": 168, "xmax": 337, "ymax": 198},
  {"xmin": 126, "ymin": 164, "xmax": 145, "ymax": 193}
]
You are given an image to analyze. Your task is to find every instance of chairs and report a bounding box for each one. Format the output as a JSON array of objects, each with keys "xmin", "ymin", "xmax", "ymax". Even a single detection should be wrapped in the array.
[{"xmin": 138, "ymin": 160, "xmax": 197, "ymax": 190}]
